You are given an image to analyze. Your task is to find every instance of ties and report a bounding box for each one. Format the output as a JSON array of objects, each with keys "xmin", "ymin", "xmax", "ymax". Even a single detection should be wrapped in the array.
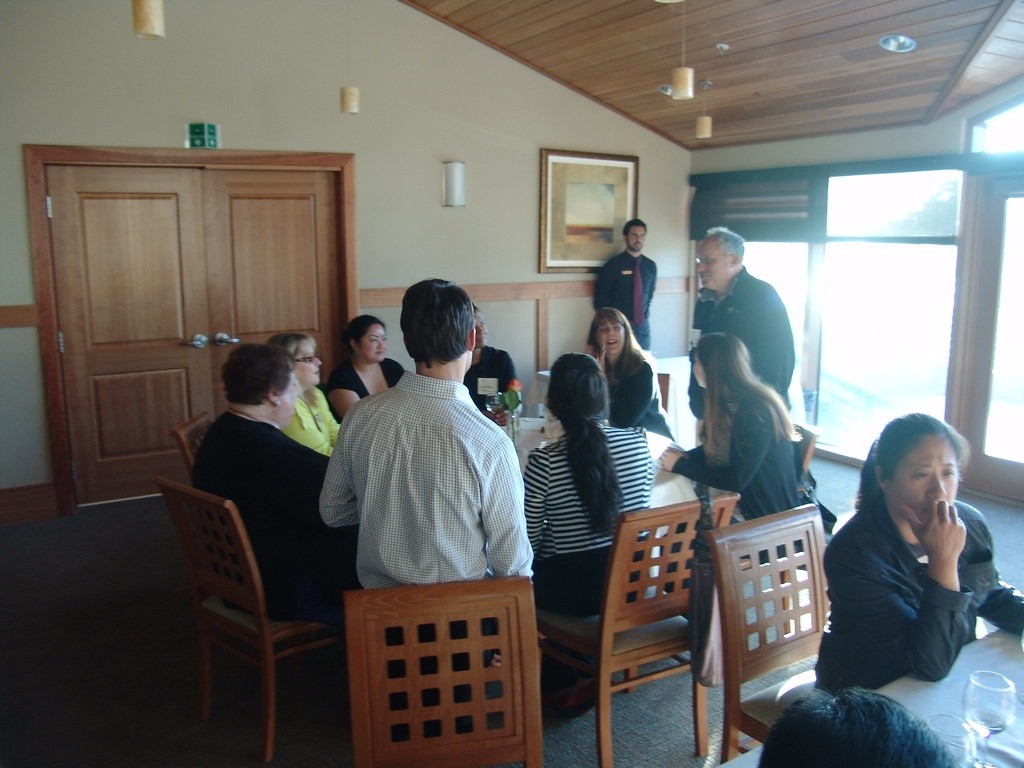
[{"xmin": 633, "ymin": 258, "xmax": 642, "ymax": 327}]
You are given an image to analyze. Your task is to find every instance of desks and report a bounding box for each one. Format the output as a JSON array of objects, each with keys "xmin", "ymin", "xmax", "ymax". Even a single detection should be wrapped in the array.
[
  {"xmin": 721, "ymin": 628, "xmax": 1024, "ymax": 768},
  {"xmin": 499, "ymin": 416, "xmax": 696, "ymax": 598}
]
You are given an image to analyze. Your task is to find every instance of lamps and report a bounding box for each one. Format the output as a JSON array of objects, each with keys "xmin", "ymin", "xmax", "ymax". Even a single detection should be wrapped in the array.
[
  {"xmin": 672, "ymin": 0, "xmax": 695, "ymax": 101},
  {"xmin": 134, "ymin": 0, "xmax": 163, "ymax": 39},
  {"xmin": 697, "ymin": 80, "xmax": 712, "ymax": 139},
  {"xmin": 442, "ymin": 160, "xmax": 464, "ymax": 208},
  {"xmin": 339, "ymin": 0, "xmax": 361, "ymax": 114}
]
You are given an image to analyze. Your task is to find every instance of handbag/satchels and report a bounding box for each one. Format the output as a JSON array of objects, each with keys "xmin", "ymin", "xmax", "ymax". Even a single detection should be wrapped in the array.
[{"xmin": 688, "ymin": 498, "xmax": 724, "ymax": 690}]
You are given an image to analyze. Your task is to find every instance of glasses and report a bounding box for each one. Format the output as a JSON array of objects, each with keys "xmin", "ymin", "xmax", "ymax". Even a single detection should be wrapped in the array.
[
  {"xmin": 294, "ymin": 356, "xmax": 323, "ymax": 364},
  {"xmin": 695, "ymin": 256, "xmax": 718, "ymax": 264}
]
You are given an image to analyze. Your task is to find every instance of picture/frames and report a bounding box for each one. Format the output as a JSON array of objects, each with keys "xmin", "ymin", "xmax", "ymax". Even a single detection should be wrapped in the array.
[{"xmin": 540, "ymin": 148, "xmax": 641, "ymax": 274}]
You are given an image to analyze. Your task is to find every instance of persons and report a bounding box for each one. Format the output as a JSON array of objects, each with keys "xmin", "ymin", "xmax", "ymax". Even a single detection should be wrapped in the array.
[
  {"xmin": 814, "ymin": 413, "xmax": 1024, "ymax": 696},
  {"xmin": 688, "ymin": 227, "xmax": 796, "ymax": 440},
  {"xmin": 192, "ymin": 303, "xmax": 799, "ymax": 714},
  {"xmin": 593, "ymin": 219, "xmax": 657, "ymax": 352},
  {"xmin": 758, "ymin": 688, "xmax": 963, "ymax": 768},
  {"xmin": 321, "ymin": 280, "xmax": 534, "ymax": 739}
]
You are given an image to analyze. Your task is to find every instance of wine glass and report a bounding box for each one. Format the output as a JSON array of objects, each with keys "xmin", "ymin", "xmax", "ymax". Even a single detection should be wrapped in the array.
[{"xmin": 962, "ymin": 670, "xmax": 1017, "ymax": 768}]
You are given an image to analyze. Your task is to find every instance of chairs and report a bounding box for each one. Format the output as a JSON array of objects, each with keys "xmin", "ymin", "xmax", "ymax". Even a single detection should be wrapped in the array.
[{"xmin": 162, "ymin": 410, "xmax": 837, "ymax": 767}]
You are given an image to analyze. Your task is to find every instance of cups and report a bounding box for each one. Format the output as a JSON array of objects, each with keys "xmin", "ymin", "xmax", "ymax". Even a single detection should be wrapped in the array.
[
  {"xmin": 925, "ymin": 713, "xmax": 977, "ymax": 768},
  {"xmin": 485, "ymin": 391, "xmax": 503, "ymax": 415}
]
